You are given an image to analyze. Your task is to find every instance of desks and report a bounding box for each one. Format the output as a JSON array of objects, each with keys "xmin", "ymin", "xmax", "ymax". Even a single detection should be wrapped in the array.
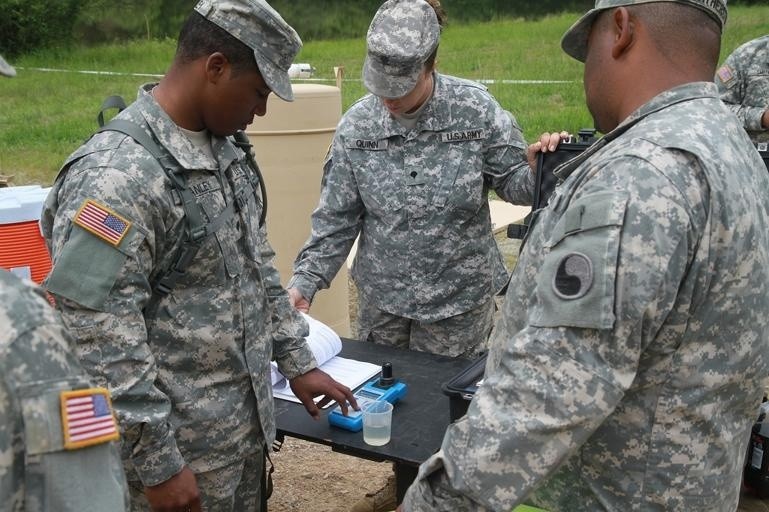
[{"xmin": 275, "ymin": 337, "xmax": 477, "ymax": 508}]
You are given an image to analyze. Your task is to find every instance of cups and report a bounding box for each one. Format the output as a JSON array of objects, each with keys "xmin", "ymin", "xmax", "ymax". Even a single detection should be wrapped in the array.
[{"xmin": 360, "ymin": 399, "xmax": 393, "ymax": 447}]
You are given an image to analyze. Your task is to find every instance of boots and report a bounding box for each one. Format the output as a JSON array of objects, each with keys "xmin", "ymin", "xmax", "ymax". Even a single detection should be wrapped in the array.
[{"xmin": 353, "ymin": 474, "xmax": 406, "ymax": 511}]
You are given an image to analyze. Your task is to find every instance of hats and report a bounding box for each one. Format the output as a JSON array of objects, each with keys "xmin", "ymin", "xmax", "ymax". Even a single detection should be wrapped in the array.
[
  {"xmin": 363, "ymin": 0, "xmax": 441, "ymax": 100},
  {"xmin": 561, "ymin": 0, "xmax": 731, "ymax": 63},
  {"xmin": 192, "ymin": 2, "xmax": 304, "ymax": 103}
]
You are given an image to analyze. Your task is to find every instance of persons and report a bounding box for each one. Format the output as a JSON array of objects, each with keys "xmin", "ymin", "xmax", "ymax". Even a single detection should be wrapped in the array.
[
  {"xmin": 385, "ymin": 0, "xmax": 769, "ymax": 512},
  {"xmin": 281, "ymin": 0, "xmax": 572, "ymax": 363},
  {"xmin": 0, "ymin": 52, "xmax": 134, "ymax": 511},
  {"xmin": 711, "ymin": 33, "xmax": 768, "ymax": 174},
  {"xmin": 36, "ymin": 1, "xmax": 366, "ymax": 511}
]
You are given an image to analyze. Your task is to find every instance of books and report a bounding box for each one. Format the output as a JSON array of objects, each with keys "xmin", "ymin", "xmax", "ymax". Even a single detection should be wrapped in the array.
[{"xmin": 264, "ymin": 311, "xmax": 383, "ymax": 411}]
